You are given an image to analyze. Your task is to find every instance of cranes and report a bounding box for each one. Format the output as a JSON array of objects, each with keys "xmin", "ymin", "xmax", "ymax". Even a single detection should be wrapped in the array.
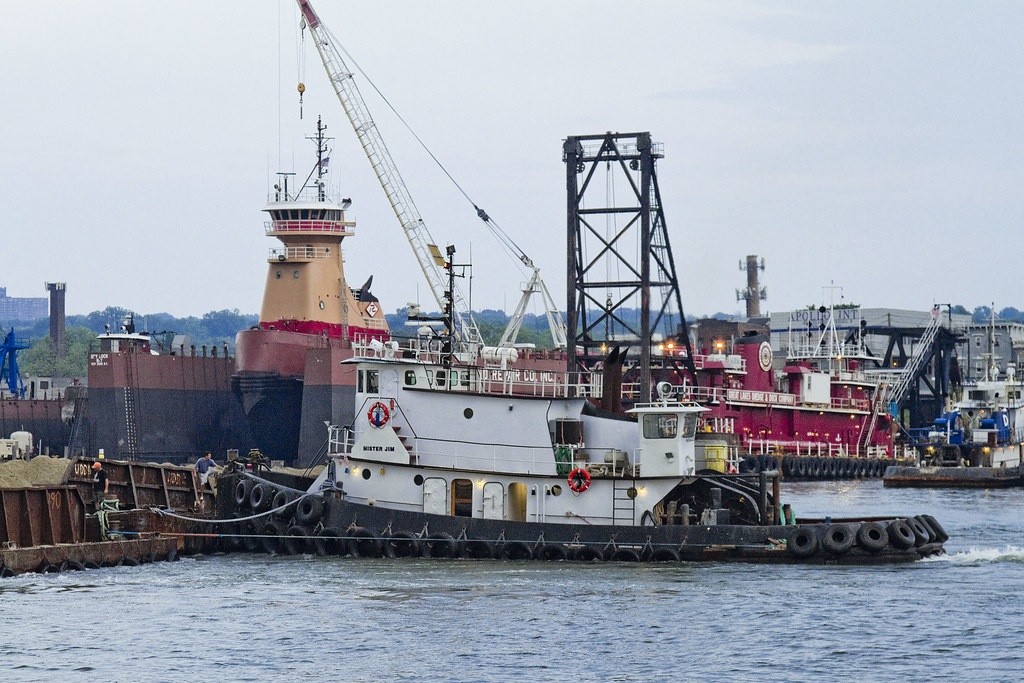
[{"xmin": 294, "ymin": 2, "xmax": 585, "ymax": 371}]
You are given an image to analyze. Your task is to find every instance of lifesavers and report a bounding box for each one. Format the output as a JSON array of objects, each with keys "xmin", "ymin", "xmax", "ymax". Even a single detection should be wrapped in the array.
[
  {"xmin": 184, "ymin": 524, "xmax": 223, "ymax": 556},
  {"xmin": 740, "ymin": 453, "xmax": 915, "ymax": 479},
  {"xmin": 224, "ymin": 512, "xmax": 685, "ymax": 563},
  {"xmin": 233, "ymin": 479, "xmax": 325, "ymax": 525},
  {"xmin": 0, "ymin": 549, "xmax": 183, "ymax": 578},
  {"xmin": 785, "ymin": 515, "xmax": 949, "ymax": 559},
  {"xmin": 567, "ymin": 468, "xmax": 592, "ymax": 493},
  {"xmin": 367, "ymin": 402, "xmax": 389, "ymax": 426},
  {"xmin": 1002, "ymin": 414, "xmax": 1009, "ymax": 427}
]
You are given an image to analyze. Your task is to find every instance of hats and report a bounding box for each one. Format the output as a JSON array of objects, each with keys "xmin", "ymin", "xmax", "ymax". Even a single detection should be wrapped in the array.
[{"xmin": 92, "ymin": 462, "xmax": 101, "ymax": 469}]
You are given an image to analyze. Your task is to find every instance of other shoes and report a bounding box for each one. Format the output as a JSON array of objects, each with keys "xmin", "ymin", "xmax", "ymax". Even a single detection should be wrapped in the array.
[
  {"xmin": 200, "ymin": 495, "xmax": 204, "ymax": 501},
  {"xmin": 93, "ymin": 512, "xmax": 98, "ymax": 515}
]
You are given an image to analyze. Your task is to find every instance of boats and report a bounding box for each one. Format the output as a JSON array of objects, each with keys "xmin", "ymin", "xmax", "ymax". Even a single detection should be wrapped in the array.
[
  {"xmin": 213, "ymin": 241, "xmax": 949, "ymax": 567},
  {"xmin": 0, "ymin": 1, "xmax": 922, "ymax": 483},
  {"xmin": 883, "ymin": 301, "xmax": 1024, "ymax": 489}
]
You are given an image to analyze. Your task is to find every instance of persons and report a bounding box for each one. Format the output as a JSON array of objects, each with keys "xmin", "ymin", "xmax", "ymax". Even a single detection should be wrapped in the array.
[
  {"xmin": 91, "ymin": 462, "xmax": 109, "ymax": 512},
  {"xmin": 525, "ymin": 348, "xmax": 529, "ymax": 359},
  {"xmin": 194, "ymin": 451, "xmax": 223, "ymax": 501},
  {"xmin": 652, "ymin": 386, "xmax": 658, "ymax": 402},
  {"xmin": 519, "ymin": 349, "xmax": 525, "ymax": 359}
]
